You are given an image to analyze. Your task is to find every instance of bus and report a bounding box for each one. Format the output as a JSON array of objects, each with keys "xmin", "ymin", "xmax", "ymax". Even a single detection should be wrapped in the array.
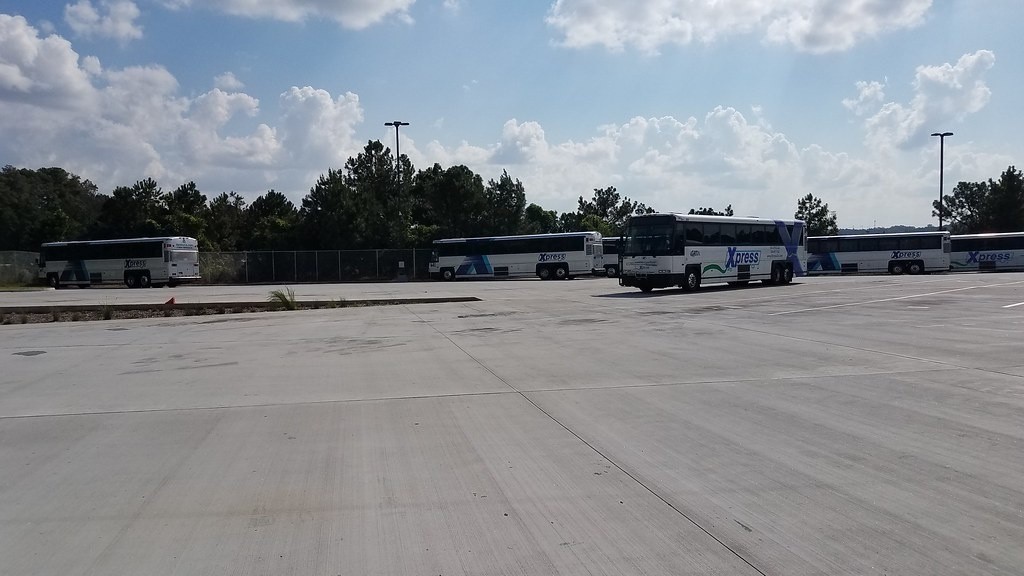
[
  {"xmin": 593, "ymin": 236, "xmax": 669, "ymax": 278},
  {"xmin": 427, "ymin": 231, "xmax": 606, "ymax": 282},
  {"xmin": 35, "ymin": 236, "xmax": 202, "ymax": 289},
  {"xmin": 806, "ymin": 231, "xmax": 950, "ymax": 275},
  {"xmin": 617, "ymin": 212, "xmax": 809, "ymax": 292},
  {"xmin": 950, "ymin": 232, "xmax": 1024, "ymax": 271}
]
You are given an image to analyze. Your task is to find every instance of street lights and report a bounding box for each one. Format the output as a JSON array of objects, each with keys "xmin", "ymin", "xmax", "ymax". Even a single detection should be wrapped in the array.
[
  {"xmin": 931, "ymin": 132, "xmax": 953, "ymax": 231},
  {"xmin": 384, "ymin": 121, "xmax": 409, "ymax": 279}
]
